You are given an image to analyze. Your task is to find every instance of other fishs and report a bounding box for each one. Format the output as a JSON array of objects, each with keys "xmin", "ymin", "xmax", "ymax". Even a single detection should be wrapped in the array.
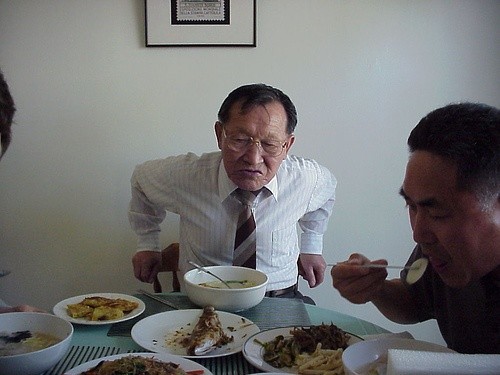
[{"xmin": 187, "ymin": 303, "xmax": 225, "ymax": 357}]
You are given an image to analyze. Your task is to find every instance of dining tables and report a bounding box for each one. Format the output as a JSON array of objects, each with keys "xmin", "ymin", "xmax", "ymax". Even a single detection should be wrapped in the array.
[{"xmin": 41, "ymin": 292, "xmax": 394, "ymax": 375}]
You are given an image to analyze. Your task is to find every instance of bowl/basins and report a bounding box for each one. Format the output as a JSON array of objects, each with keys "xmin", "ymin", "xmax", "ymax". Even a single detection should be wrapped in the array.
[
  {"xmin": 183, "ymin": 266, "xmax": 268, "ymax": 313},
  {"xmin": 0, "ymin": 312, "xmax": 73, "ymax": 375},
  {"xmin": 341, "ymin": 338, "xmax": 458, "ymax": 375}
]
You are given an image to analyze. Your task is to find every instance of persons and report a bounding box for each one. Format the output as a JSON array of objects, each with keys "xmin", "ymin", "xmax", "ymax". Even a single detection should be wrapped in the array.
[
  {"xmin": 330, "ymin": 103, "xmax": 500, "ymax": 354},
  {"xmin": 131, "ymin": 84, "xmax": 339, "ymax": 305}
]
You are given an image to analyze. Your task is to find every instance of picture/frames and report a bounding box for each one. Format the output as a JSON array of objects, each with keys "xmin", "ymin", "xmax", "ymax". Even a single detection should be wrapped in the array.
[{"xmin": 144, "ymin": 0, "xmax": 256, "ymax": 48}]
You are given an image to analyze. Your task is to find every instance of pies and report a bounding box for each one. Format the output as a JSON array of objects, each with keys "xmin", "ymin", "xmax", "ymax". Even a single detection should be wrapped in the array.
[{"xmin": 66, "ymin": 296, "xmax": 139, "ymax": 322}]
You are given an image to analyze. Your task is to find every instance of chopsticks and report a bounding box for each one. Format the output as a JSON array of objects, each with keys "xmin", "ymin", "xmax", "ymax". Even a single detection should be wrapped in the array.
[{"xmin": 136, "ymin": 289, "xmax": 181, "ymax": 310}]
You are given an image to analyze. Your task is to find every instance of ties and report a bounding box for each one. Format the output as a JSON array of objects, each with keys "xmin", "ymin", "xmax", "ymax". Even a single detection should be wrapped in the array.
[{"xmin": 230, "ymin": 188, "xmax": 264, "ymax": 270}]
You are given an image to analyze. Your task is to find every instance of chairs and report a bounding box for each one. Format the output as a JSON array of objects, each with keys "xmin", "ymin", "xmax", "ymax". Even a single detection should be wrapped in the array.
[{"xmin": 153, "ymin": 243, "xmax": 318, "ymax": 292}]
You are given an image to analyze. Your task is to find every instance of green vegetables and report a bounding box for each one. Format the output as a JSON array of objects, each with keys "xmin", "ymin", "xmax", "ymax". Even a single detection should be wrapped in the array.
[
  {"xmin": 254, "ymin": 334, "xmax": 295, "ymax": 367},
  {"xmin": 199, "ymin": 280, "xmax": 247, "ymax": 288}
]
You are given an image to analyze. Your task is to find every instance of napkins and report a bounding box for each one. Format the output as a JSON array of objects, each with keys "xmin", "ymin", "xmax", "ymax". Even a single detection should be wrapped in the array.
[{"xmin": 360, "ymin": 331, "xmax": 414, "ymax": 342}]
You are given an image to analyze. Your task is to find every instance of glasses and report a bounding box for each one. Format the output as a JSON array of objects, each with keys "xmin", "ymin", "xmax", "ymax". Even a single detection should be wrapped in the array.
[{"xmin": 222, "ymin": 127, "xmax": 288, "ymax": 157}]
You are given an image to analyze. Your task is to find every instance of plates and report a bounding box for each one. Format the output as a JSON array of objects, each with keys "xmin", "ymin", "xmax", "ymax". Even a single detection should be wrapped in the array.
[
  {"xmin": 53, "ymin": 292, "xmax": 146, "ymax": 325},
  {"xmin": 242, "ymin": 326, "xmax": 365, "ymax": 374},
  {"xmin": 131, "ymin": 309, "xmax": 261, "ymax": 359},
  {"xmin": 63, "ymin": 352, "xmax": 213, "ymax": 375}
]
